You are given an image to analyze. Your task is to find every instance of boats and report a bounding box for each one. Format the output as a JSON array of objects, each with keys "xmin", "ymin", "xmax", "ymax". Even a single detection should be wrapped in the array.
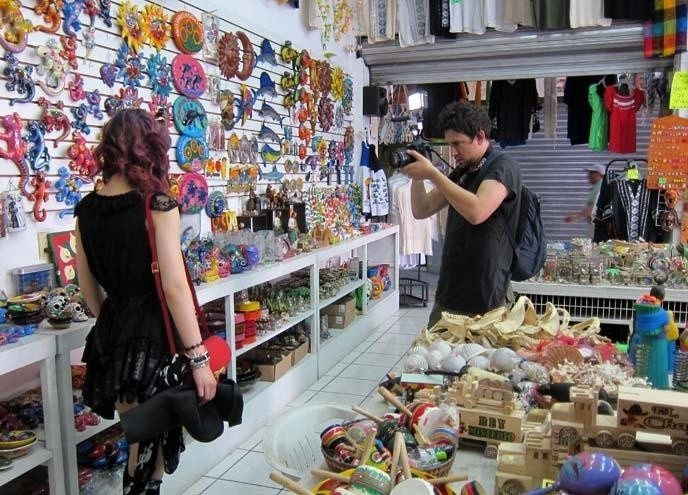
[{"xmin": 363, "ymin": 86, "xmax": 389, "ymax": 118}]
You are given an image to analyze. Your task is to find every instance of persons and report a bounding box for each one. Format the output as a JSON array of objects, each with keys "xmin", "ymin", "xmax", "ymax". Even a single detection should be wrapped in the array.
[
  {"xmin": 563, "ymin": 163, "xmax": 608, "ymax": 243},
  {"xmin": 73, "ymin": 105, "xmax": 222, "ymax": 494},
  {"xmin": 395, "ymin": 99, "xmax": 525, "ymax": 331}
]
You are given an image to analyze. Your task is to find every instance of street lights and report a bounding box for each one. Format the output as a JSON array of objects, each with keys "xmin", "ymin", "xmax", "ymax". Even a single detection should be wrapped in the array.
[{"xmin": 179, "ymin": 335, "xmax": 231, "ymax": 384}]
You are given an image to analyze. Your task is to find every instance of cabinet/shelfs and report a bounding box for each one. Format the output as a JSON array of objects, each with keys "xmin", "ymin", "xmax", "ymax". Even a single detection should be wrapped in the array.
[
  {"xmin": 34, "ymin": 221, "xmax": 403, "ymax": 495},
  {"xmin": 0, "ymin": 329, "xmax": 66, "ymax": 495}
]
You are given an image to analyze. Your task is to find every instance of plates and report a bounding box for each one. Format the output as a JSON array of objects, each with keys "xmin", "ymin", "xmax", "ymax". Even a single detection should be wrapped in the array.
[
  {"xmin": 0, "ymin": 329, "xmax": 66, "ymax": 495},
  {"xmin": 34, "ymin": 221, "xmax": 403, "ymax": 495}
]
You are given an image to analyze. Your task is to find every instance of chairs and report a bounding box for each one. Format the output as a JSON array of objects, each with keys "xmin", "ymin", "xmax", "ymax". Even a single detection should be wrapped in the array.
[{"xmin": 263, "ymin": 403, "xmax": 358, "ymax": 479}]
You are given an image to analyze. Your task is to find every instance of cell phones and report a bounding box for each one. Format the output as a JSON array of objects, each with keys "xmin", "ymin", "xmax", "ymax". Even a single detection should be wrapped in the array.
[{"xmin": 390, "ymin": 140, "xmax": 433, "ymax": 171}]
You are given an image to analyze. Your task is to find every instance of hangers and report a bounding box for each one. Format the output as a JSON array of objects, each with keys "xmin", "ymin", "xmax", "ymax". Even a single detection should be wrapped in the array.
[
  {"xmin": 619, "ymin": 161, "xmax": 643, "ymax": 182},
  {"xmin": 594, "ymin": 72, "xmax": 635, "ymax": 94}
]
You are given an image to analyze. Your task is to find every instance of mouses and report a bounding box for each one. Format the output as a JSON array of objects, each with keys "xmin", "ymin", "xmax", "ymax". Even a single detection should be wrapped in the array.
[
  {"xmin": 594, "ymin": 72, "xmax": 635, "ymax": 94},
  {"xmin": 619, "ymin": 161, "xmax": 643, "ymax": 182}
]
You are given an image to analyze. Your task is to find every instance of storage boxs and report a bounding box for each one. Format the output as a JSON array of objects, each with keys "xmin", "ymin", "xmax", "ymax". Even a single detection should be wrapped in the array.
[
  {"xmin": 320, "ymin": 296, "xmax": 357, "ymax": 329},
  {"xmin": 243, "ymin": 340, "xmax": 309, "ymax": 382}
]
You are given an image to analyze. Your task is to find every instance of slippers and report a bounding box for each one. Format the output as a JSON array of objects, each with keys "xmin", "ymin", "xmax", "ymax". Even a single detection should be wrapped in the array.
[{"xmin": 475, "ymin": 151, "xmax": 547, "ymax": 282}]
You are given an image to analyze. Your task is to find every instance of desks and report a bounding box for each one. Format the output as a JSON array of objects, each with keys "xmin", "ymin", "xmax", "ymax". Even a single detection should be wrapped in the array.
[{"xmin": 312, "ymin": 268, "xmax": 687, "ymax": 494}]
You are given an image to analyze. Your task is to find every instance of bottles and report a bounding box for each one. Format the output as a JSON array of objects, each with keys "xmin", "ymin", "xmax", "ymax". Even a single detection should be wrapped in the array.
[{"xmin": 584, "ymin": 165, "xmax": 606, "ymax": 176}]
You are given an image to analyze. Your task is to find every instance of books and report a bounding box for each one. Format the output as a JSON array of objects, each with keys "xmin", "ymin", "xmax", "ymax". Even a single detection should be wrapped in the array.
[
  {"xmin": 320, "ymin": 296, "xmax": 357, "ymax": 329},
  {"xmin": 243, "ymin": 340, "xmax": 309, "ymax": 382}
]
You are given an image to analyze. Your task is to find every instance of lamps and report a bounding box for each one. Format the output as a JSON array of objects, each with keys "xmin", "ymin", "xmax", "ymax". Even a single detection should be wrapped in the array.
[{"xmin": 263, "ymin": 403, "xmax": 358, "ymax": 479}]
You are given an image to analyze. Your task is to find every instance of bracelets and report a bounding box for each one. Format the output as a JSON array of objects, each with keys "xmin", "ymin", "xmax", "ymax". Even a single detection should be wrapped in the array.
[
  {"xmin": 184, "ymin": 340, "xmax": 205, "ymax": 354},
  {"xmin": 183, "ymin": 350, "xmax": 210, "ymax": 370}
]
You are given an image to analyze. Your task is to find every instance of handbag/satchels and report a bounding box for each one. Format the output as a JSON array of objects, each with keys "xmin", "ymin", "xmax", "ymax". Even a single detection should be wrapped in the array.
[
  {"xmin": 184, "ymin": 340, "xmax": 205, "ymax": 354},
  {"xmin": 183, "ymin": 350, "xmax": 210, "ymax": 370}
]
